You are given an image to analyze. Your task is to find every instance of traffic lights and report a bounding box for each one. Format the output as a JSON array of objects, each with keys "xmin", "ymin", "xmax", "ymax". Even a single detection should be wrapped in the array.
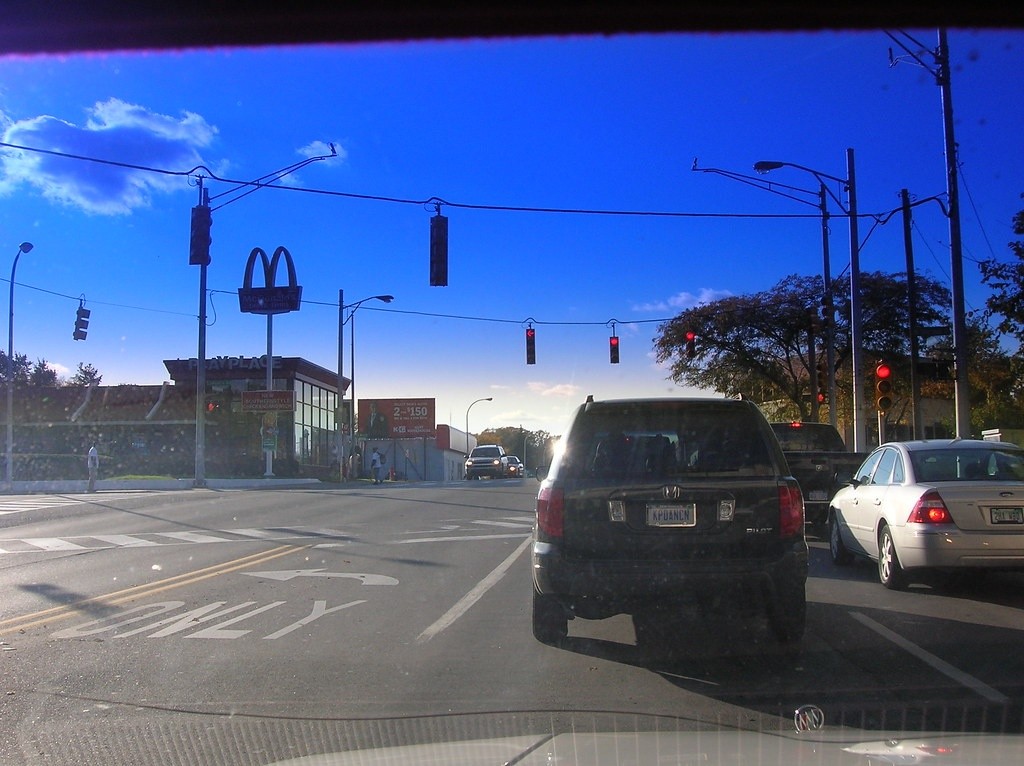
[
  {"xmin": 817, "ymin": 362, "xmax": 829, "ymax": 389},
  {"xmin": 190, "ymin": 205, "xmax": 214, "ymax": 266},
  {"xmin": 73, "ymin": 307, "xmax": 91, "ymax": 340},
  {"xmin": 820, "ymin": 295, "xmax": 835, "ymax": 329},
  {"xmin": 525, "ymin": 329, "xmax": 535, "ymax": 364},
  {"xmin": 205, "ymin": 398, "xmax": 221, "ymax": 417},
  {"xmin": 685, "ymin": 330, "xmax": 696, "ymax": 358},
  {"xmin": 610, "ymin": 336, "xmax": 619, "ymax": 364},
  {"xmin": 874, "ymin": 363, "xmax": 894, "ymax": 411},
  {"xmin": 817, "ymin": 391, "xmax": 826, "ymax": 404},
  {"xmin": 805, "ymin": 302, "xmax": 823, "ymax": 337}
]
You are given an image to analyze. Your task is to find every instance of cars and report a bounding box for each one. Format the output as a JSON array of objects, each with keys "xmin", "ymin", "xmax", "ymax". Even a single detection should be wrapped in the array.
[
  {"xmin": 506, "ymin": 455, "xmax": 524, "ymax": 479},
  {"xmin": 827, "ymin": 437, "xmax": 1024, "ymax": 590}
]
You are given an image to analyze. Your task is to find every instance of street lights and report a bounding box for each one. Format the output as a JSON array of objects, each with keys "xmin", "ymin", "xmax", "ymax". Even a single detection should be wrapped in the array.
[
  {"xmin": 753, "ymin": 148, "xmax": 867, "ymax": 453},
  {"xmin": 6, "ymin": 241, "xmax": 34, "ymax": 490},
  {"xmin": 466, "ymin": 398, "xmax": 493, "ymax": 456},
  {"xmin": 335, "ymin": 288, "xmax": 395, "ymax": 483}
]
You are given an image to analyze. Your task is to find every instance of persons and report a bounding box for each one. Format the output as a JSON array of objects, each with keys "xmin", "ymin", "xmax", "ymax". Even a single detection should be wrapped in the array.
[
  {"xmin": 370, "ymin": 447, "xmax": 384, "ymax": 485},
  {"xmin": 88, "ymin": 442, "xmax": 100, "ymax": 492},
  {"xmin": 348, "ymin": 453, "xmax": 361, "ymax": 478},
  {"xmin": 365, "ymin": 401, "xmax": 388, "ymax": 439}
]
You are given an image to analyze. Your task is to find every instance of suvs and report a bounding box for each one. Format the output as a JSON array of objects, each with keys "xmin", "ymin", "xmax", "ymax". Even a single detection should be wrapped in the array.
[
  {"xmin": 531, "ymin": 393, "xmax": 810, "ymax": 647},
  {"xmin": 464, "ymin": 444, "xmax": 509, "ymax": 480},
  {"xmin": 766, "ymin": 420, "xmax": 851, "ymax": 542}
]
